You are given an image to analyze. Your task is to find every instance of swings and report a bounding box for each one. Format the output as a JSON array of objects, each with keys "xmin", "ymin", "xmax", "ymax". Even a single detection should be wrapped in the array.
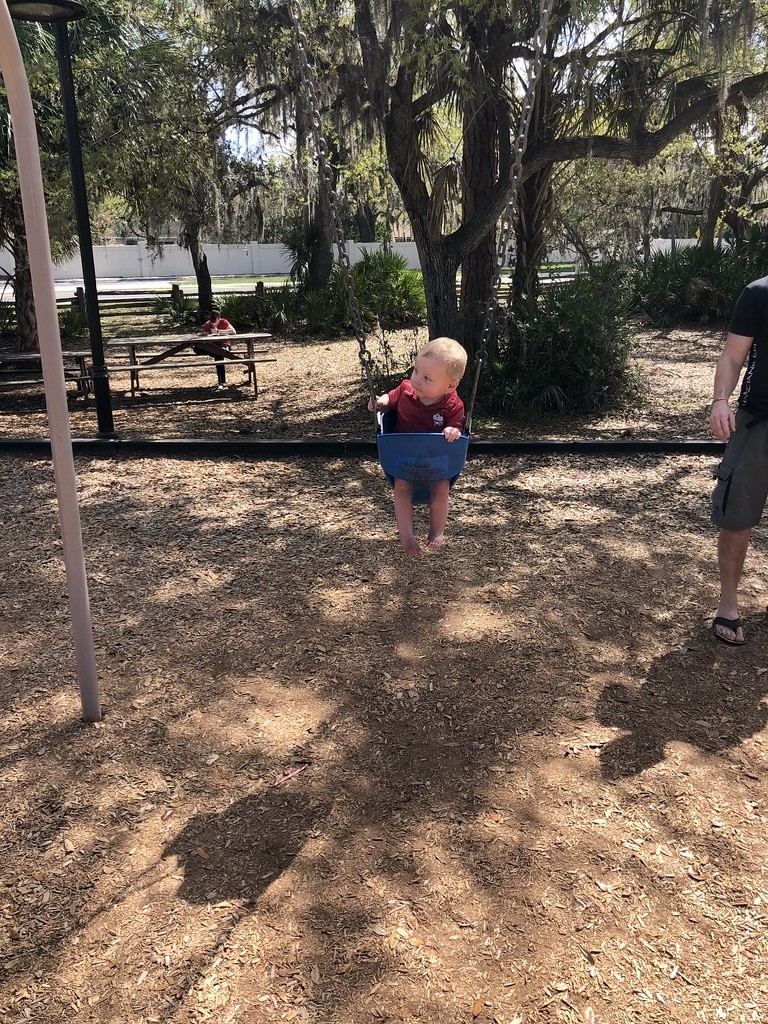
[{"xmin": 281, "ymin": 1, "xmax": 550, "ymax": 503}]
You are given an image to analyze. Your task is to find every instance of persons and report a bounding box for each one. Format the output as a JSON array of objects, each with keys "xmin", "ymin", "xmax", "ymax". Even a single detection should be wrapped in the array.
[
  {"xmin": 198, "ymin": 310, "xmax": 249, "ymax": 390},
  {"xmin": 367, "ymin": 336, "xmax": 468, "ymax": 558},
  {"xmin": 707, "ymin": 271, "xmax": 768, "ymax": 645}
]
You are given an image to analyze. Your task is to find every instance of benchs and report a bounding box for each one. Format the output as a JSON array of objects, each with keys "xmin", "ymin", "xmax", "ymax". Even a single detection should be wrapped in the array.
[
  {"xmin": 112, "ymin": 350, "xmax": 268, "ymax": 388},
  {"xmin": 106, "ymin": 357, "xmax": 278, "ymax": 407},
  {"xmin": 0, "ymin": 376, "xmax": 91, "ymax": 404},
  {"xmin": 0, "ymin": 367, "xmax": 92, "ymax": 392}
]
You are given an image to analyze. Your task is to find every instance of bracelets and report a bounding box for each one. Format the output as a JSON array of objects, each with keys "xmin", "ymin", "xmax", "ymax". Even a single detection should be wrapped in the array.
[
  {"xmin": 217, "ymin": 329, "xmax": 220, "ymax": 333},
  {"xmin": 710, "ymin": 396, "xmax": 729, "ymax": 409}
]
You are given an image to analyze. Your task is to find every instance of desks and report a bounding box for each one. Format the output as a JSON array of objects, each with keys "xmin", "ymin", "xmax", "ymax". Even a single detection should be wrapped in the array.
[
  {"xmin": 0, "ymin": 348, "xmax": 92, "ymax": 404},
  {"xmin": 107, "ymin": 332, "xmax": 273, "ymax": 406}
]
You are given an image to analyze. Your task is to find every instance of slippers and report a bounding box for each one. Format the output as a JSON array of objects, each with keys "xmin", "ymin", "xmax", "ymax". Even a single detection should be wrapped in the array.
[{"xmin": 713, "ymin": 616, "xmax": 747, "ymax": 646}]
